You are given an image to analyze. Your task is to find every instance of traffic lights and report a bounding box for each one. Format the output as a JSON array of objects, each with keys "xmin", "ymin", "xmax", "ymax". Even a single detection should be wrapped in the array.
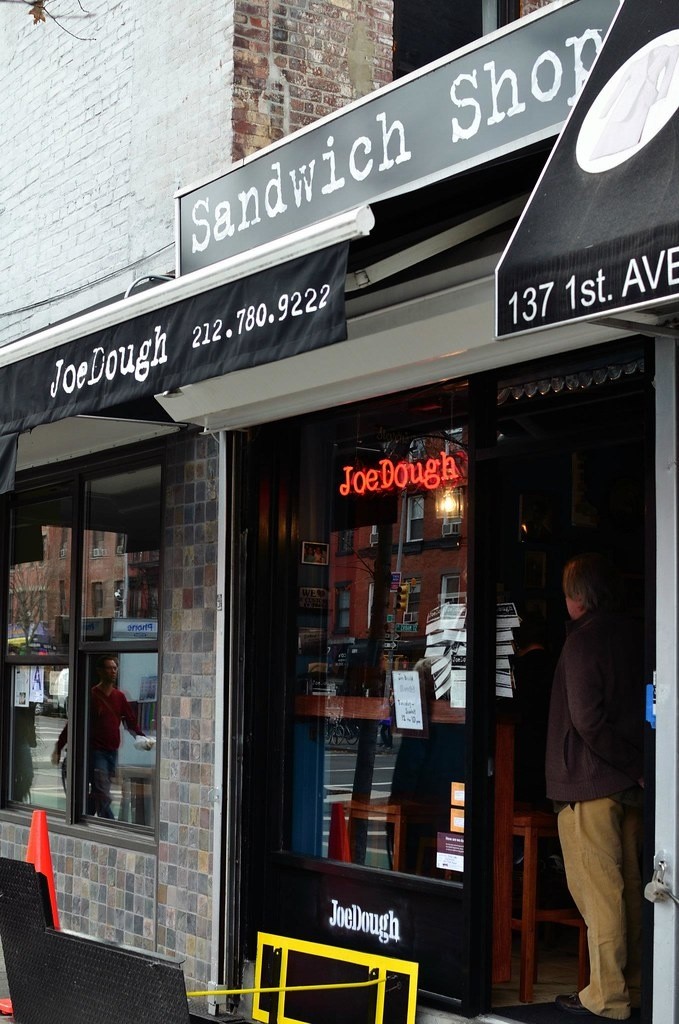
[{"xmin": 396, "ymin": 584, "xmax": 409, "ymax": 611}]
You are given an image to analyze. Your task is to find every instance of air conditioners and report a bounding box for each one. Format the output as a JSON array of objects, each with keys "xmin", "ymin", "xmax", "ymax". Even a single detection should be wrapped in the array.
[
  {"xmin": 404, "ymin": 612, "xmax": 417, "ymax": 622},
  {"xmin": 368, "ymin": 533, "xmax": 379, "ymax": 544},
  {"xmin": 441, "ymin": 522, "xmax": 459, "ymax": 536}
]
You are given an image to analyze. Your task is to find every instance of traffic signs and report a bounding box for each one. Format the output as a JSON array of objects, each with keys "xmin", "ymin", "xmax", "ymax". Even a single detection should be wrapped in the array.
[
  {"xmin": 383, "ymin": 641, "xmax": 397, "ymax": 650},
  {"xmin": 384, "ymin": 632, "xmax": 401, "ymax": 641}
]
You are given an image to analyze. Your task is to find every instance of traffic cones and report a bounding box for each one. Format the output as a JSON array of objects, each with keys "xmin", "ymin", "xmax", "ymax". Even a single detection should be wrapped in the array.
[
  {"xmin": 0, "ymin": 809, "xmax": 64, "ymax": 1016},
  {"xmin": 327, "ymin": 803, "xmax": 350, "ymax": 862}
]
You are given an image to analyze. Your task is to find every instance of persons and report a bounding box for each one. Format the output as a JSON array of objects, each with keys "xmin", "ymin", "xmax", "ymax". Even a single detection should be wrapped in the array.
[
  {"xmin": 544, "ymin": 554, "xmax": 645, "ymax": 1020},
  {"xmin": 52, "ymin": 656, "xmax": 153, "ymax": 818},
  {"xmin": 380, "ymin": 655, "xmax": 458, "ymax": 880}
]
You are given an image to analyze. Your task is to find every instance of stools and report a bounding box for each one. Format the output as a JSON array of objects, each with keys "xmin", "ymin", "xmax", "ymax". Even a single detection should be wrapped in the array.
[
  {"xmin": 508, "ymin": 798, "xmax": 593, "ymax": 1004},
  {"xmin": 342, "ymin": 793, "xmax": 446, "ymax": 876}
]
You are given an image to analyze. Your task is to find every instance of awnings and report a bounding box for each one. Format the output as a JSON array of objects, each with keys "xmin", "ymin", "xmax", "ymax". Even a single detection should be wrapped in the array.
[
  {"xmin": 0, "ymin": 203, "xmax": 377, "ymax": 495},
  {"xmin": 496, "ymin": 0, "xmax": 679, "ymax": 341}
]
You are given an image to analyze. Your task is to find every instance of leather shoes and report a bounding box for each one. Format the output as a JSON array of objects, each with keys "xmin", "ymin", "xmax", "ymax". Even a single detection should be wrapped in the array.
[{"xmin": 556, "ymin": 992, "xmax": 595, "ymax": 1015}]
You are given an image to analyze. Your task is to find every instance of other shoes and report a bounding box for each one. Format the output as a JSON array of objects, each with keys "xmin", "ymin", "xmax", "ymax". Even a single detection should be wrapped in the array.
[
  {"xmin": 382, "ymin": 744, "xmax": 389, "ymax": 747},
  {"xmin": 387, "ymin": 746, "xmax": 392, "ymax": 750}
]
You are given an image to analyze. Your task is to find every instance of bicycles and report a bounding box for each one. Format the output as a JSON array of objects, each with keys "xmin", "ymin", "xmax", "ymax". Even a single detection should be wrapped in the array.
[{"xmin": 323, "ymin": 710, "xmax": 360, "ymax": 746}]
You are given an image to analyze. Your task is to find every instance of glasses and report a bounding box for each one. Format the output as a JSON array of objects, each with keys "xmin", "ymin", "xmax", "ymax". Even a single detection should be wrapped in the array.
[{"xmin": 104, "ymin": 666, "xmax": 118, "ymax": 672}]
